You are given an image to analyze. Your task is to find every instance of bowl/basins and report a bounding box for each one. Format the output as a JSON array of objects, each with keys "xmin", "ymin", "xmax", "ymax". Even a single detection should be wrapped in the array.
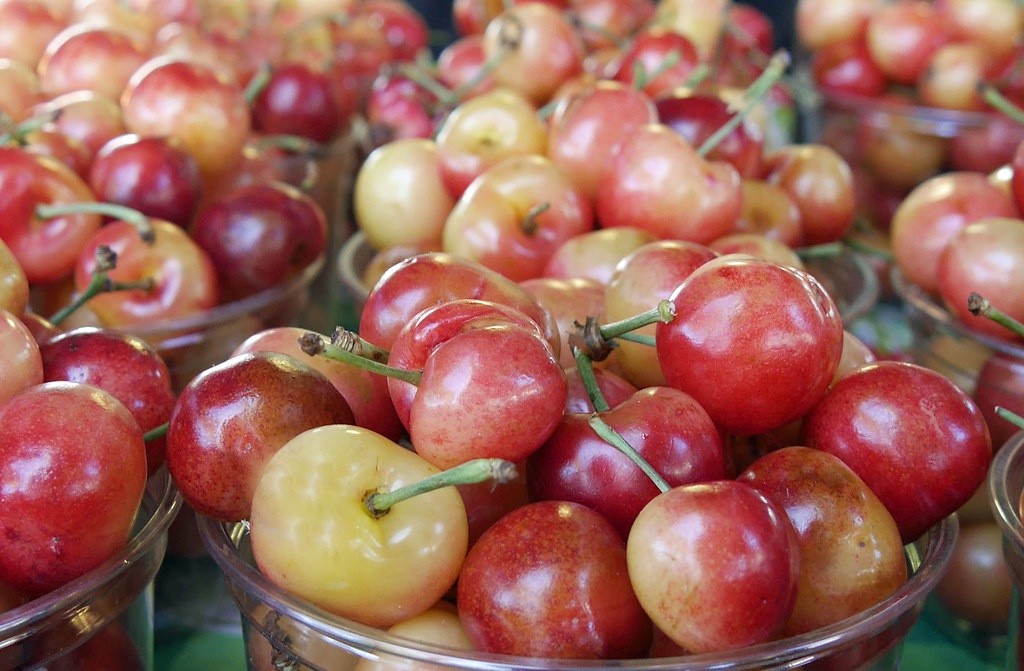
[
  {"xmin": 792, "ymin": 61, "xmax": 1024, "ymax": 233},
  {"xmin": 889, "ymin": 262, "xmax": 1024, "ymax": 455},
  {"xmin": 340, "ymin": 234, "xmax": 882, "ymax": 362},
  {"xmin": 100, "ymin": 249, "xmax": 327, "ymax": 403},
  {"xmin": 235, "ymin": 130, "xmax": 355, "ymax": 248},
  {"xmin": 0, "ymin": 462, "xmax": 183, "ymax": 671},
  {"xmin": 986, "ymin": 429, "xmax": 1024, "ymax": 591},
  {"xmin": 193, "ymin": 508, "xmax": 958, "ymax": 671}
]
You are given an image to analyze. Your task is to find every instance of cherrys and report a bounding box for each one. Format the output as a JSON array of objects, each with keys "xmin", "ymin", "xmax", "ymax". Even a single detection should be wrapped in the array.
[{"xmin": 0, "ymin": 0, "xmax": 1024, "ymax": 671}]
